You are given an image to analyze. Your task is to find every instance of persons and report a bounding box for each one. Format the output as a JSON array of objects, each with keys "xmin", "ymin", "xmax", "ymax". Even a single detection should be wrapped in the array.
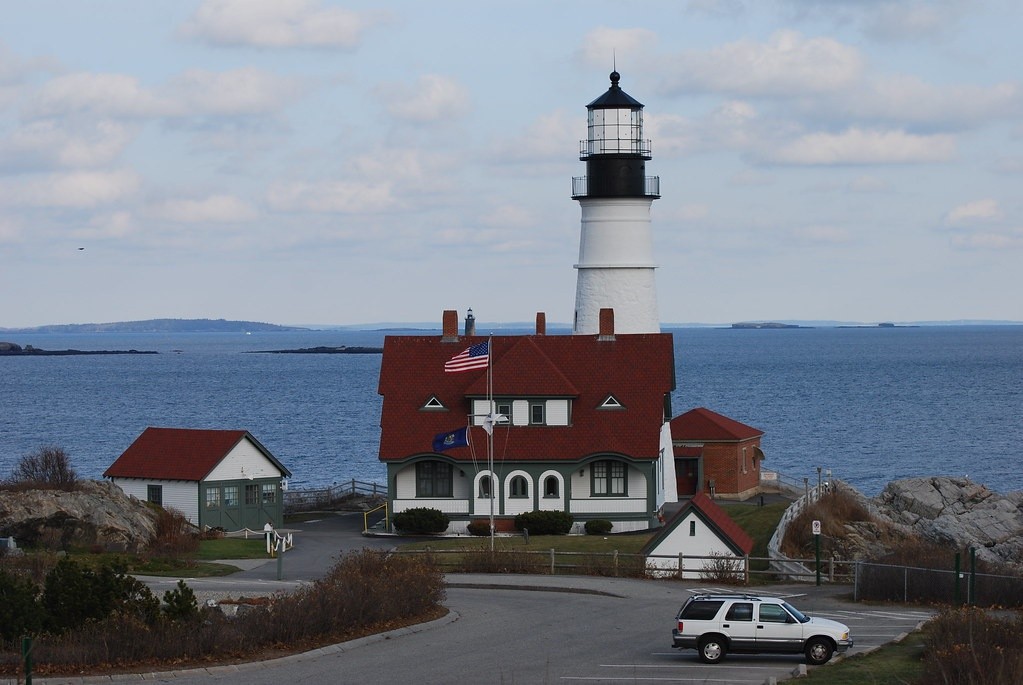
[{"xmin": 263, "ymin": 520, "xmax": 274, "ymax": 539}]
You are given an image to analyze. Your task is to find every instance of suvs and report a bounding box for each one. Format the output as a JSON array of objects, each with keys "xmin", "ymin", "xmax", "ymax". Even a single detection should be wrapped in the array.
[{"xmin": 672, "ymin": 592, "xmax": 854, "ymax": 663}]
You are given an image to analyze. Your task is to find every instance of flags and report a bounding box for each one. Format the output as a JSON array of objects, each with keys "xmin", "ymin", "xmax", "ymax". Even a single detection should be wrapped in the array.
[
  {"xmin": 431, "ymin": 424, "xmax": 470, "ymax": 454},
  {"xmin": 445, "ymin": 339, "xmax": 489, "ymax": 372}
]
